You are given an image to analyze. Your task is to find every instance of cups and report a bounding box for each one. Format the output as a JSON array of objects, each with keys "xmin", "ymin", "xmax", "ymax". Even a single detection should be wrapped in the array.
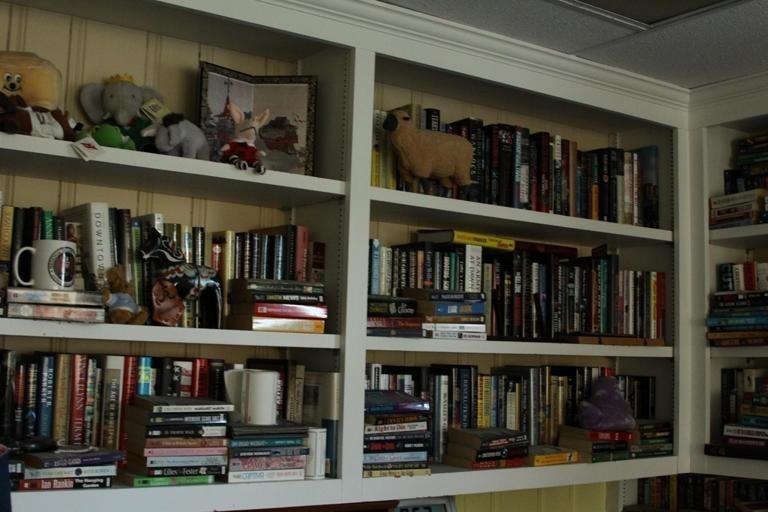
[{"xmin": 14, "ymin": 239, "xmax": 78, "ymax": 291}]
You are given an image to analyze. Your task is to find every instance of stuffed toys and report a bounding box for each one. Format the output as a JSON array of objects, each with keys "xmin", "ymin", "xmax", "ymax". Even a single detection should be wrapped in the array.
[{"xmin": 0, "ymin": 49, "xmax": 322, "ymax": 178}]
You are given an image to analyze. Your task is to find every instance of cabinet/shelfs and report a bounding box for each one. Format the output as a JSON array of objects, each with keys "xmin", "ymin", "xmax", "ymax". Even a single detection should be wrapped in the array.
[
  {"xmin": 691, "ymin": 70, "xmax": 768, "ymax": 480},
  {"xmin": 296, "ymin": 1, "xmax": 691, "ymax": 504},
  {"xmin": 1, "ymin": 1, "xmax": 376, "ymax": 511}
]
